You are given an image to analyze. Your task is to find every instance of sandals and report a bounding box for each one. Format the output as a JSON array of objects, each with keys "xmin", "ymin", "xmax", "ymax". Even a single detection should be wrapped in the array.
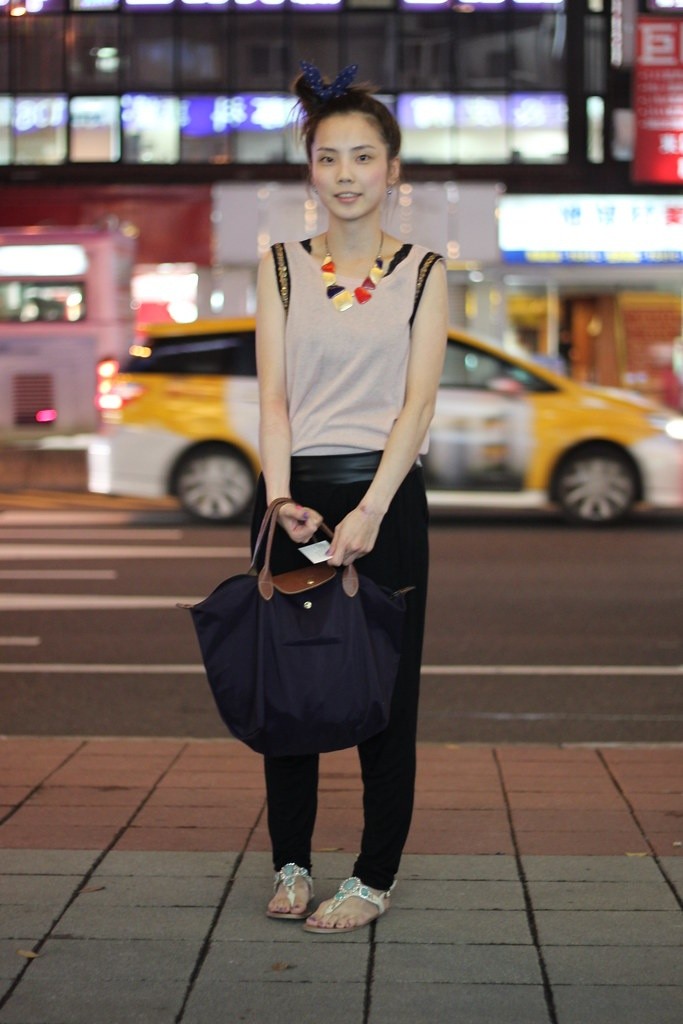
[
  {"xmin": 265, "ymin": 863, "xmax": 315, "ymax": 920},
  {"xmin": 302, "ymin": 876, "xmax": 398, "ymax": 933}
]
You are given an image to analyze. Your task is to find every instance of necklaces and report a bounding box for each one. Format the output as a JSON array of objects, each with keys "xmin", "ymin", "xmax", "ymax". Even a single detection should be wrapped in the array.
[{"xmin": 322, "ymin": 227, "xmax": 385, "ymax": 312}]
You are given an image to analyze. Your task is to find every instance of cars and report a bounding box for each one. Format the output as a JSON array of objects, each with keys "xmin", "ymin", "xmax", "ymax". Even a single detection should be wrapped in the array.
[{"xmin": 93, "ymin": 316, "xmax": 683, "ymax": 525}]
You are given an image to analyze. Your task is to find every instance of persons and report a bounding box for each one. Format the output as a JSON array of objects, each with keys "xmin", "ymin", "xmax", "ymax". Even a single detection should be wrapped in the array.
[{"xmin": 249, "ymin": 62, "xmax": 451, "ymax": 933}]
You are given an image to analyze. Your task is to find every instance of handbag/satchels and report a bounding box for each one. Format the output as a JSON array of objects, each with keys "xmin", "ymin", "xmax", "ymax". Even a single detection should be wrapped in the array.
[{"xmin": 175, "ymin": 496, "xmax": 375, "ymax": 756}]
